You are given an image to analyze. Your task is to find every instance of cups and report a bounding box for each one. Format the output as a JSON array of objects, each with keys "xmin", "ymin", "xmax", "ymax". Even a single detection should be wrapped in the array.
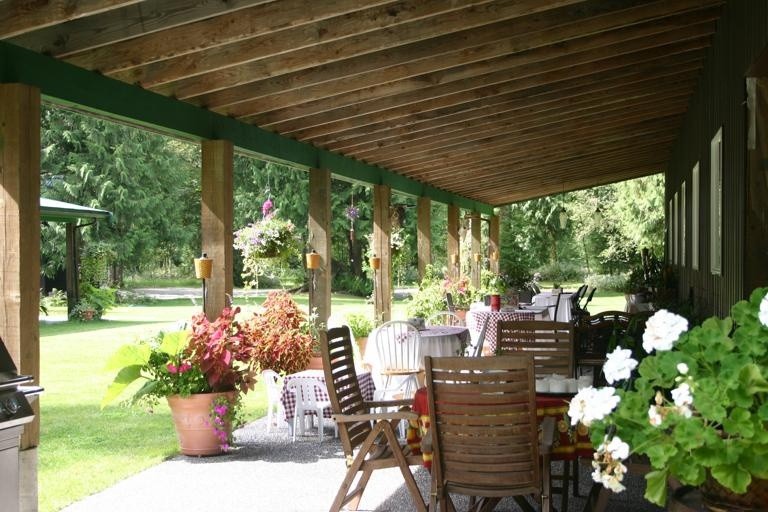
[{"xmin": 490, "ymin": 295, "xmax": 500, "ymax": 312}]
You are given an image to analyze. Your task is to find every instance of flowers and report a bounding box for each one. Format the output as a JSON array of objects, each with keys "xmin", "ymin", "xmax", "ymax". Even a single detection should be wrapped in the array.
[
  {"xmin": 566, "ymin": 287, "xmax": 767, "ymax": 508},
  {"xmin": 232, "ymin": 211, "xmax": 305, "ymax": 301},
  {"xmin": 91, "ymin": 294, "xmax": 266, "ymax": 453}
]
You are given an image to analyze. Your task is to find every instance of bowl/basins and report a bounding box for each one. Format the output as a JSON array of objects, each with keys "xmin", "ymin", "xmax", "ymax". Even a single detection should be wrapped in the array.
[{"xmin": 519, "ymin": 303, "xmax": 532, "ymax": 309}]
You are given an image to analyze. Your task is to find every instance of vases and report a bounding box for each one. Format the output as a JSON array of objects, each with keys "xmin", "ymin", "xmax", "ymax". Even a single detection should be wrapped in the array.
[
  {"xmin": 700, "ymin": 463, "xmax": 767, "ymax": 512},
  {"xmin": 258, "ymin": 242, "xmax": 276, "ymax": 259},
  {"xmin": 165, "ymin": 392, "xmax": 238, "ymax": 458}
]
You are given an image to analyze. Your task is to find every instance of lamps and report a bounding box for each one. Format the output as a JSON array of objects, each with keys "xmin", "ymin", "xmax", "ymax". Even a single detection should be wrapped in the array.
[
  {"xmin": 193, "ymin": 253, "xmax": 212, "ymax": 313},
  {"xmin": 305, "ymin": 249, "xmax": 320, "ymax": 316},
  {"xmin": 369, "ymin": 253, "xmax": 379, "ymax": 327}
]
